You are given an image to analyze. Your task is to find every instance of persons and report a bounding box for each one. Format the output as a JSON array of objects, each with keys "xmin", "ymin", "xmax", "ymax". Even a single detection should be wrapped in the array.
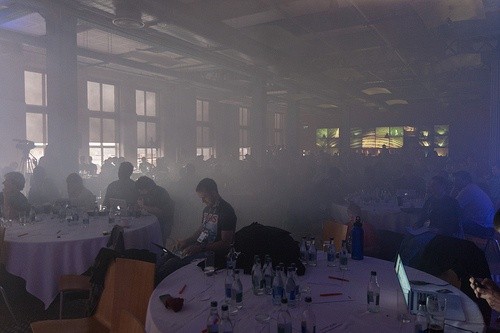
[
  {"xmin": 310, "ymin": 144, "xmax": 500, "ymax": 333},
  {"xmin": 178, "ymin": 154, "xmax": 304, "ymax": 177},
  {"xmin": 153, "ymin": 177, "xmax": 237, "ymax": 290},
  {"xmin": 0, "ymin": 154, "xmax": 176, "ymax": 267}
]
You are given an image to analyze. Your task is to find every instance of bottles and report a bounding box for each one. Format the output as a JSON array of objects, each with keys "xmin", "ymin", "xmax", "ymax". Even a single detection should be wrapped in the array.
[
  {"xmin": 339, "ymin": 239, "xmax": 349, "ymax": 271},
  {"xmin": 366, "ymin": 270, "xmax": 380, "ymax": 313},
  {"xmin": 301, "ymin": 296, "xmax": 315, "ymax": 333},
  {"xmin": 327, "ymin": 237, "xmax": 336, "ymax": 267},
  {"xmin": 225, "ymin": 264, "xmax": 234, "ymax": 304},
  {"xmin": 231, "ymin": 270, "xmax": 243, "ymax": 306},
  {"xmin": 218, "ymin": 304, "xmax": 233, "ymax": 333},
  {"xmin": 207, "ymin": 301, "xmax": 221, "ymax": 333},
  {"xmin": 300, "ymin": 236, "xmax": 317, "ymax": 266},
  {"xmin": 277, "ymin": 298, "xmax": 292, "ymax": 333},
  {"xmin": 251, "ymin": 254, "xmax": 301, "ymax": 310}
]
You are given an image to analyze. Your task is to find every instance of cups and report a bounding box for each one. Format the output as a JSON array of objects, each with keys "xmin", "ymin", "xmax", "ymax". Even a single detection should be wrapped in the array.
[
  {"xmin": 397, "ymin": 288, "xmax": 447, "ymax": 333},
  {"xmin": 5, "ymin": 199, "xmax": 143, "ymax": 228}
]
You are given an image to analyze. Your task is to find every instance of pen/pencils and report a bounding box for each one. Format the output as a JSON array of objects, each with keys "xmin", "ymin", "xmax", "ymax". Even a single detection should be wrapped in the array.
[
  {"xmin": 328, "ymin": 276, "xmax": 349, "ymax": 282},
  {"xmin": 179, "ymin": 284, "xmax": 187, "ymax": 294},
  {"xmin": 320, "ymin": 292, "xmax": 343, "ymax": 296}
]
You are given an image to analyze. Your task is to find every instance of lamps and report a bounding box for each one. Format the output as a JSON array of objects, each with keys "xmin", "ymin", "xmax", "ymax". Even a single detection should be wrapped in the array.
[{"xmin": 112, "ymin": 5, "xmax": 145, "ymax": 30}]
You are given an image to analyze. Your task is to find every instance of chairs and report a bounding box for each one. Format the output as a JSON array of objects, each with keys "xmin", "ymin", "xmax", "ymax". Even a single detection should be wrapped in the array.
[
  {"xmin": 0, "ymin": 220, "xmax": 156, "ymax": 333},
  {"xmin": 397, "ymin": 208, "xmax": 431, "ymax": 237},
  {"xmin": 323, "ymin": 221, "xmax": 349, "ymax": 254},
  {"xmin": 398, "ymin": 232, "xmax": 492, "ymax": 329}
]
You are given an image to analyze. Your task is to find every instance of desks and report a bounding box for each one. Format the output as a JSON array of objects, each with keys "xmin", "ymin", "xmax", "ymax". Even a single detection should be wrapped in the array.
[
  {"xmin": 145, "ymin": 251, "xmax": 486, "ymax": 333},
  {"xmin": 0, "ymin": 213, "xmax": 165, "ymax": 311},
  {"xmin": 331, "ymin": 189, "xmax": 431, "ymax": 237}
]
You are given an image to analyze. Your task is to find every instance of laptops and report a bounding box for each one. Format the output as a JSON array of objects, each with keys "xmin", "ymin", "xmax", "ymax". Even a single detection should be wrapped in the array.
[
  {"xmin": 392, "ymin": 251, "xmax": 467, "ymax": 321},
  {"xmin": 152, "ymin": 241, "xmax": 190, "ymax": 261}
]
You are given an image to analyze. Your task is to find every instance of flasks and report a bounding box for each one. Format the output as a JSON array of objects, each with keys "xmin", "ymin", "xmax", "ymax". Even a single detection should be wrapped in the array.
[{"xmin": 350, "ymin": 215, "xmax": 364, "ymax": 260}]
